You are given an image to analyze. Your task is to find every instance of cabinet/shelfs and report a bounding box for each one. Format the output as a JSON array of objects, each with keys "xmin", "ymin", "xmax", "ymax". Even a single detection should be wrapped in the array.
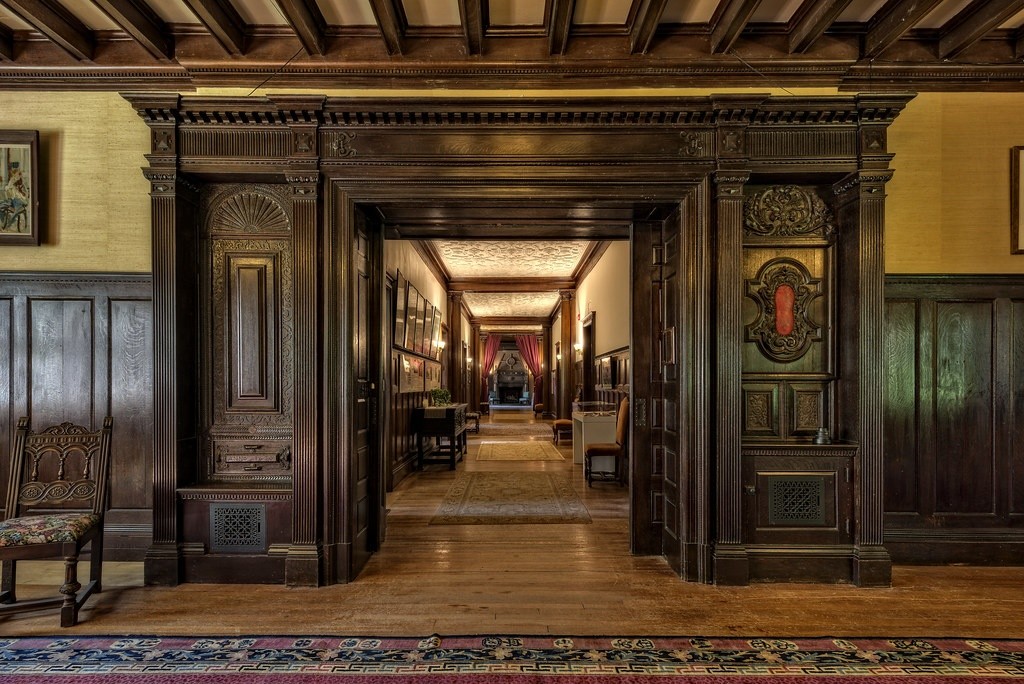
[{"xmin": 416, "ymin": 403, "xmax": 469, "ymax": 471}]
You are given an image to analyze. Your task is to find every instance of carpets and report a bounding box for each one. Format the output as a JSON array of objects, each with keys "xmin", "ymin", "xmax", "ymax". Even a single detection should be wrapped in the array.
[
  {"xmin": 0, "ymin": 633, "xmax": 1024, "ymax": 684},
  {"xmin": 428, "ymin": 470, "xmax": 593, "ymax": 524},
  {"xmin": 475, "ymin": 441, "xmax": 566, "ymax": 462}
]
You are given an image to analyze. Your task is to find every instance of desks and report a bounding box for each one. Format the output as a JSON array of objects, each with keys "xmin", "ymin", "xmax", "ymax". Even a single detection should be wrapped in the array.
[{"xmin": 571, "ymin": 401, "xmax": 617, "ymax": 476}]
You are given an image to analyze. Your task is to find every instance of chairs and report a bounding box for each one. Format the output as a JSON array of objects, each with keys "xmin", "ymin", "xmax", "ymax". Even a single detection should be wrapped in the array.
[
  {"xmin": 553, "ymin": 396, "xmax": 581, "ymax": 446},
  {"xmin": 585, "ymin": 397, "xmax": 629, "ymax": 488},
  {"xmin": 0, "ymin": 416, "xmax": 115, "ymax": 628}
]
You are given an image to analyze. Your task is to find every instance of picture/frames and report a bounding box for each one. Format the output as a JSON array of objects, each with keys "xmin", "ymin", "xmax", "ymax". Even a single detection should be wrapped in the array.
[
  {"xmin": 396, "ymin": 270, "xmax": 443, "ymax": 394},
  {"xmin": 0, "ymin": 129, "xmax": 41, "ymax": 246},
  {"xmin": 1009, "ymin": 145, "xmax": 1024, "ymax": 255}
]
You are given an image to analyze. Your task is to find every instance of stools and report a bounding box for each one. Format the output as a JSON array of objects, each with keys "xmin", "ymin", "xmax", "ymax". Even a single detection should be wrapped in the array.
[
  {"xmin": 534, "ymin": 403, "xmax": 543, "ymax": 417},
  {"xmin": 480, "ymin": 402, "xmax": 490, "ymax": 415},
  {"xmin": 466, "ymin": 413, "xmax": 479, "ymax": 434}
]
